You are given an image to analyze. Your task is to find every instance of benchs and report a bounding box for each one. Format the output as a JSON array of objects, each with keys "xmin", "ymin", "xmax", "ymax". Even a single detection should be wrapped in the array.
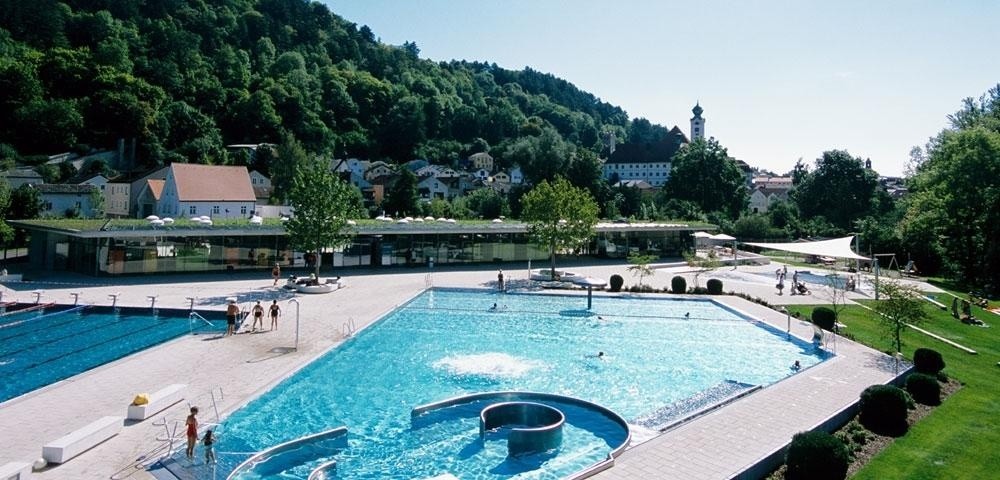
[{"xmin": 0, "ymin": 383, "xmax": 189, "ymax": 480}]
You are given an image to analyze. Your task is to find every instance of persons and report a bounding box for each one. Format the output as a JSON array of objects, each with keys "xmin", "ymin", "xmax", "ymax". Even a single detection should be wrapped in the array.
[
  {"xmin": 685, "ymin": 312, "xmax": 690, "ymax": 317},
  {"xmin": 271, "ymin": 262, "xmax": 280, "ymax": 285},
  {"xmin": 200, "ymin": 430, "xmax": 217, "ymax": 465},
  {"xmin": 304, "ymin": 252, "xmax": 316, "ymax": 273},
  {"xmin": 268, "ymin": 300, "xmax": 281, "ymax": 331},
  {"xmin": 248, "ymin": 248, "xmax": 254, "ymax": 265},
  {"xmin": 952, "ymin": 291, "xmax": 989, "ymax": 325},
  {"xmin": 405, "ymin": 247, "xmax": 417, "ymax": 266},
  {"xmin": 731, "ymin": 246, "xmax": 735, "ymax": 254},
  {"xmin": 490, "ymin": 302, "xmax": 497, "ymax": 309},
  {"xmin": 724, "ymin": 248, "xmax": 727, "ymax": 252},
  {"xmin": 226, "ymin": 299, "xmax": 239, "ymax": 336},
  {"xmin": 850, "ymin": 275, "xmax": 855, "ymax": 291},
  {"xmin": 252, "ymin": 301, "xmax": 264, "ymax": 331},
  {"xmin": 775, "ymin": 264, "xmax": 808, "ymax": 296},
  {"xmin": 0, "ymin": 264, "xmax": 7, "ymax": 277},
  {"xmin": 795, "ymin": 360, "xmax": 801, "ymax": 370},
  {"xmin": 597, "ymin": 316, "xmax": 602, "ymax": 320},
  {"xmin": 590, "ymin": 351, "xmax": 604, "ymax": 361},
  {"xmin": 498, "ymin": 269, "xmax": 504, "ymax": 292},
  {"xmin": 185, "ymin": 407, "xmax": 200, "ymax": 457}
]
